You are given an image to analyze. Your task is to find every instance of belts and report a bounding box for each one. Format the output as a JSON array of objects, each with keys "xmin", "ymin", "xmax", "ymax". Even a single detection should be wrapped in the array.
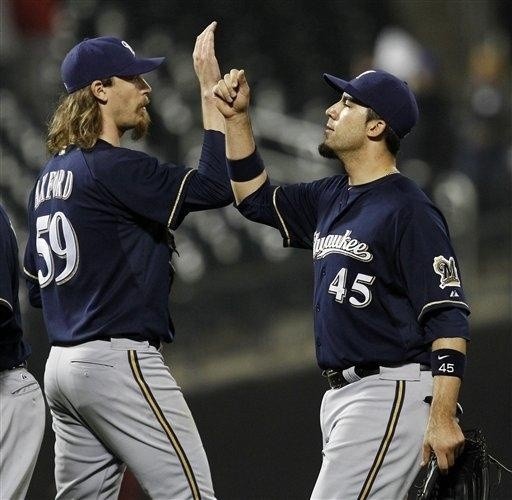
[{"xmin": 327, "ymin": 363, "xmax": 380, "ymax": 389}]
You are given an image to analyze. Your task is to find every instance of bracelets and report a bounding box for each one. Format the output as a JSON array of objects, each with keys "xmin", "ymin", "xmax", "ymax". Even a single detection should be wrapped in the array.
[{"xmin": 431, "ymin": 349, "xmax": 466, "ymax": 383}]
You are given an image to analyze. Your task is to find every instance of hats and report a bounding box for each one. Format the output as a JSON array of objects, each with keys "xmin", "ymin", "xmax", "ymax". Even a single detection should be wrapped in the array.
[
  {"xmin": 60, "ymin": 37, "xmax": 166, "ymax": 94},
  {"xmin": 322, "ymin": 69, "xmax": 420, "ymax": 139}
]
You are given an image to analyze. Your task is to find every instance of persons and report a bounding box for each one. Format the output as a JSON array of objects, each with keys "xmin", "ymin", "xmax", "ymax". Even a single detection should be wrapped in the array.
[
  {"xmin": 0, "ymin": 22, "xmax": 471, "ymax": 287},
  {"xmin": 24, "ymin": 19, "xmax": 233, "ymax": 500},
  {"xmin": 211, "ymin": 69, "xmax": 470, "ymax": 500},
  {"xmin": 0, "ymin": 209, "xmax": 47, "ymax": 500}
]
